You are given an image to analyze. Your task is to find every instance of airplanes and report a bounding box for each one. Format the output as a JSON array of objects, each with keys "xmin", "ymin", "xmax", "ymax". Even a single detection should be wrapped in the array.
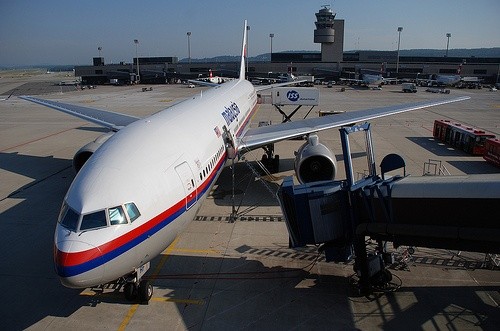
[{"xmin": 19, "ymin": 17, "xmax": 473, "ymax": 307}]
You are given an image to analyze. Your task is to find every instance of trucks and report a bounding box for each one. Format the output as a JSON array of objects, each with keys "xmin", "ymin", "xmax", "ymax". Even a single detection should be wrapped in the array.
[{"xmin": 434, "ymin": 118, "xmax": 500, "ymax": 168}]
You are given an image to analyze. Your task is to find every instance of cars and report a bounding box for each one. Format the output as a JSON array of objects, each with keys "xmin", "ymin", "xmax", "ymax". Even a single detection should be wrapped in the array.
[{"xmin": 321, "ymin": 72, "xmax": 500, "ymax": 95}]
